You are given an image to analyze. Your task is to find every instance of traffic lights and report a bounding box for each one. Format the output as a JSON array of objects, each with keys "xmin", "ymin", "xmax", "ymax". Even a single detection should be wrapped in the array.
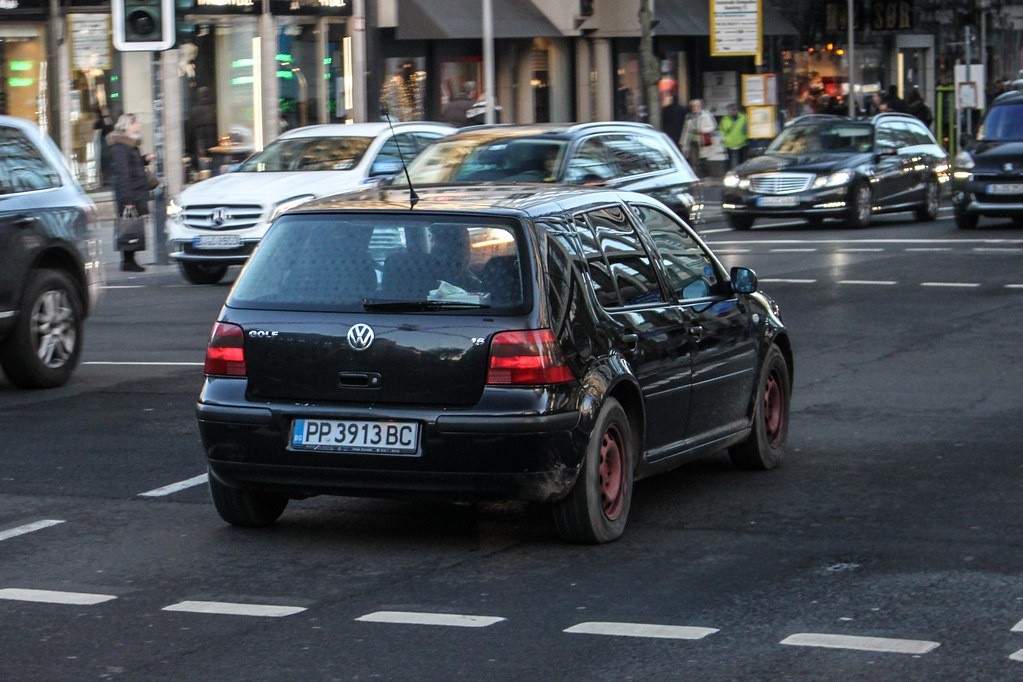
[{"xmin": 110, "ymin": 0, "xmax": 176, "ymax": 52}]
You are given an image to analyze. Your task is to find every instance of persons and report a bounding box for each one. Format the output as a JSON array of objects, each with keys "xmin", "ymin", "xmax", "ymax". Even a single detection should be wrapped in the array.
[
  {"xmin": 675, "ymin": 84, "xmax": 934, "ymax": 178},
  {"xmin": 428, "ymin": 224, "xmax": 482, "ymax": 291},
  {"xmin": 105, "ymin": 113, "xmax": 156, "ymax": 272},
  {"xmin": 178, "ymin": 43, "xmax": 209, "ymax": 181},
  {"xmin": 541, "ymin": 149, "xmax": 561, "ymax": 177}
]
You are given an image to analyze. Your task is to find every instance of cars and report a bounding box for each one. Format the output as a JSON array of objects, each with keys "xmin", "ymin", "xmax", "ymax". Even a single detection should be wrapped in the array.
[{"xmin": 196, "ymin": 183, "xmax": 797, "ymax": 546}]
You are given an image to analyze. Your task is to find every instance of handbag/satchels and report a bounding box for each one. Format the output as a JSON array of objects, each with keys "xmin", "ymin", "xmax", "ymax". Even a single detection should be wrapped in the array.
[
  {"xmin": 116, "ymin": 205, "xmax": 146, "ymax": 252},
  {"xmin": 143, "ymin": 163, "xmax": 158, "ymax": 190}
]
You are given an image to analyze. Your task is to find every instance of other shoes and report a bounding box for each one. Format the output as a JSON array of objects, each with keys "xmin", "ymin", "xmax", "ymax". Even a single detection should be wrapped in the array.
[{"xmin": 122, "ymin": 262, "xmax": 145, "ymax": 272}]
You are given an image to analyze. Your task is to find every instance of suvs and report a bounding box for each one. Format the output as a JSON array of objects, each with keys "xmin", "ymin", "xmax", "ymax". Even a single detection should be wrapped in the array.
[
  {"xmin": 951, "ymin": 78, "xmax": 1023, "ymax": 230},
  {"xmin": 0, "ymin": 115, "xmax": 103, "ymax": 392},
  {"xmin": 165, "ymin": 121, "xmax": 491, "ymax": 286},
  {"xmin": 719, "ymin": 111, "xmax": 950, "ymax": 231},
  {"xmin": 377, "ymin": 117, "xmax": 706, "ymax": 233}
]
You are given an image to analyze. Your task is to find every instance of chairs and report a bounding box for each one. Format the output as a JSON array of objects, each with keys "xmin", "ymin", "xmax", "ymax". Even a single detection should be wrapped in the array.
[
  {"xmin": 381, "ymin": 253, "xmax": 449, "ymax": 301},
  {"xmin": 294, "ymin": 241, "xmax": 378, "ymax": 296},
  {"xmin": 471, "ymin": 255, "xmax": 525, "ymax": 309},
  {"xmin": 520, "ymin": 157, "xmax": 547, "ymax": 174}
]
